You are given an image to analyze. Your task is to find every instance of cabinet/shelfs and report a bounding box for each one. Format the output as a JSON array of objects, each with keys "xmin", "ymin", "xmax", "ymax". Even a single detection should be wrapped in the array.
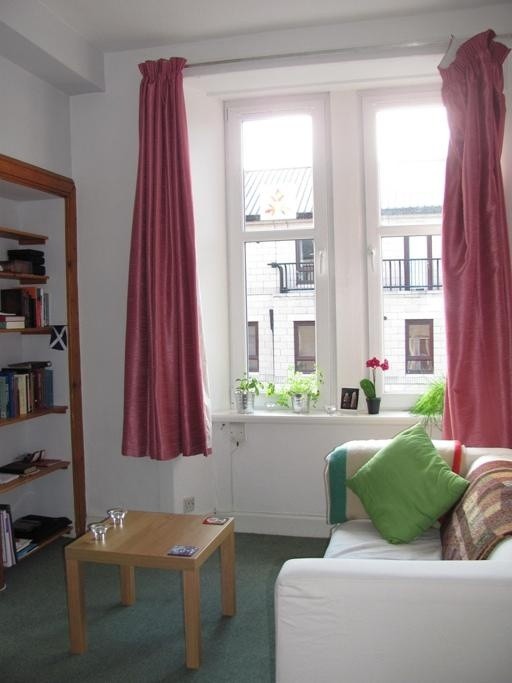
[{"xmin": 1, "ymin": 152, "xmax": 86, "ymax": 592}]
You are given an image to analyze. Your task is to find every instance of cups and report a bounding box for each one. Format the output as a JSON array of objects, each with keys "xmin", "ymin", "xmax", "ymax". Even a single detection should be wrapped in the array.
[
  {"xmin": 89, "ymin": 523, "xmax": 109, "ymax": 543},
  {"xmin": 291, "ymin": 392, "xmax": 309, "ymax": 413},
  {"xmin": 107, "ymin": 508, "xmax": 128, "ymax": 529},
  {"xmin": 235, "ymin": 390, "xmax": 255, "ymax": 414}
]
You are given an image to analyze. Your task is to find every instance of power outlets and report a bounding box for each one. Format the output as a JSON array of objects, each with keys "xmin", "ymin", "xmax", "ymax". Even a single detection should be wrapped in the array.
[{"xmin": 183, "ymin": 497, "xmax": 195, "ymax": 513}]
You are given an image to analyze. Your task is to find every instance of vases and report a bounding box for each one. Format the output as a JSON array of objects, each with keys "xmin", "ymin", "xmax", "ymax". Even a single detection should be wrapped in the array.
[{"xmin": 366, "ymin": 398, "xmax": 382, "ymax": 414}]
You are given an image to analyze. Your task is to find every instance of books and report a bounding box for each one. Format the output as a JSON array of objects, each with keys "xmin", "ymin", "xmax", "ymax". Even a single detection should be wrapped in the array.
[{"xmin": 0, "ymin": 250, "xmax": 53, "ymax": 567}]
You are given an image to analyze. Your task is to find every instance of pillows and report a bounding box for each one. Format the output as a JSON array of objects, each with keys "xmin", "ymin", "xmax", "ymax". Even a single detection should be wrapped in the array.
[{"xmin": 344, "ymin": 422, "xmax": 470, "ymax": 544}]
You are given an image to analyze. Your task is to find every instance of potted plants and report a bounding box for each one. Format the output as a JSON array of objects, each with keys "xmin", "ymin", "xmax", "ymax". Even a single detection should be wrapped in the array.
[
  {"xmin": 232, "ymin": 372, "xmax": 264, "ymax": 414},
  {"xmin": 266, "ymin": 362, "xmax": 325, "ymax": 413}
]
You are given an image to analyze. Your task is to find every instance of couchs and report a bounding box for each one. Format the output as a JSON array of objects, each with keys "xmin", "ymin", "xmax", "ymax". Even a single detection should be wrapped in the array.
[{"xmin": 274, "ymin": 438, "xmax": 512, "ymax": 683}]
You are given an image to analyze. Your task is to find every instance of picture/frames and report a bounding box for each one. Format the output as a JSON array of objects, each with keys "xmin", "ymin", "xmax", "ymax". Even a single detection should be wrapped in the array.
[{"xmin": 338, "ymin": 386, "xmax": 360, "ymax": 412}]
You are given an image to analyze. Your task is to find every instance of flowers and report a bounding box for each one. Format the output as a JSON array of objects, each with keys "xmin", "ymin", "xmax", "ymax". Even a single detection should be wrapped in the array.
[{"xmin": 360, "ymin": 356, "xmax": 389, "ymax": 398}]
